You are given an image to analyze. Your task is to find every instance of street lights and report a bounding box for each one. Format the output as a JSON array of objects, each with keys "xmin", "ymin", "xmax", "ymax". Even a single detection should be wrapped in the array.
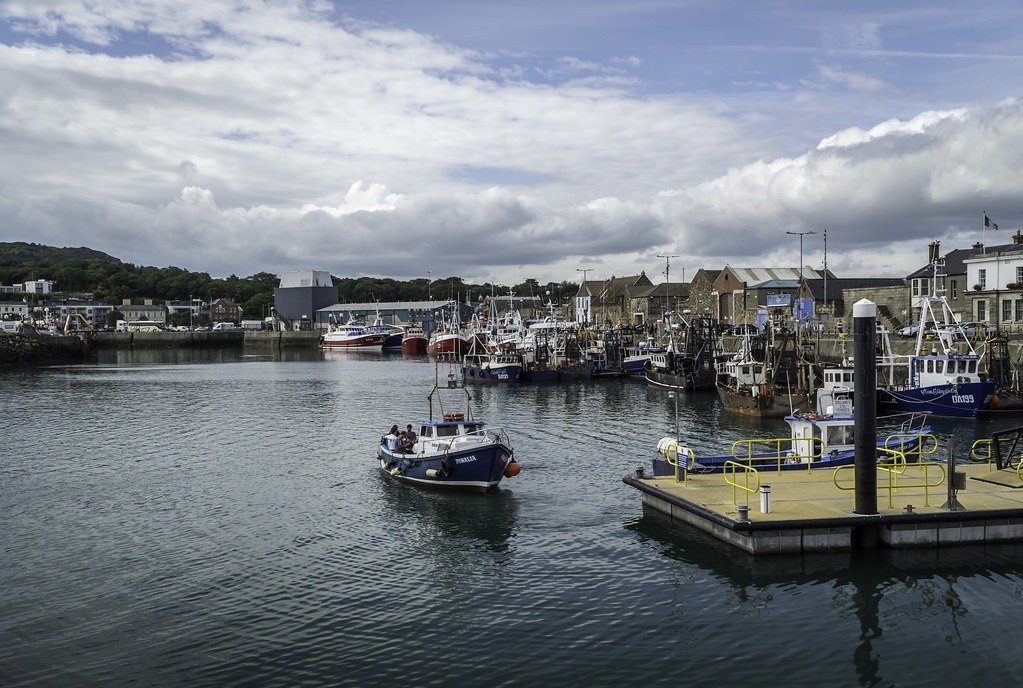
[
  {"xmin": 656, "ymin": 255, "xmax": 679, "ymax": 310},
  {"xmin": 783, "ymin": 232, "xmax": 818, "ymax": 318},
  {"xmin": 576, "ymin": 268, "xmax": 595, "ymax": 309}
]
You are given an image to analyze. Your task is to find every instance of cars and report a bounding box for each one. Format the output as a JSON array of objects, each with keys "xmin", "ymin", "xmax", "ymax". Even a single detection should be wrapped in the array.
[
  {"xmin": 726, "ymin": 323, "xmax": 759, "ymax": 335},
  {"xmin": 166, "ymin": 325, "xmax": 212, "ymax": 333},
  {"xmin": 897, "ymin": 321, "xmax": 937, "ymax": 339},
  {"xmin": 950, "ymin": 321, "xmax": 997, "ymax": 340}
]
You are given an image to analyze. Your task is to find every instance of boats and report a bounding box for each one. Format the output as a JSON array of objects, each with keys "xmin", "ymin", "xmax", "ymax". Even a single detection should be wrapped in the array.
[
  {"xmin": 652, "ymin": 385, "xmax": 934, "ymax": 476},
  {"xmin": 318, "ymin": 253, "xmax": 1002, "ymax": 421},
  {"xmin": 376, "ymin": 345, "xmax": 521, "ymax": 496}
]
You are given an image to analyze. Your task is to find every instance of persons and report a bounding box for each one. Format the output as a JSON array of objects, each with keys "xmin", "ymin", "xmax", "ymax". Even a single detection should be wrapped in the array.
[
  {"xmin": 837, "ymin": 319, "xmax": 843, "ymax": 333},
  {"xmin": 805, "ymin": 318, "xmax": 825, "ymax": 339},
  {"xmin": 384, "ymin": 424, "xmax": 418, "ymax": 453},
  {"xmin": 780, "ymin": 316, "xmax": 788, "ymax": 329}
]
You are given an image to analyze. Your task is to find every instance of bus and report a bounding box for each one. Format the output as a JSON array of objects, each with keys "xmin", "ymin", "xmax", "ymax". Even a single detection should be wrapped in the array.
[{"xmin": 128, "ymin": 320, "xmax": 167, "ymax": 333}]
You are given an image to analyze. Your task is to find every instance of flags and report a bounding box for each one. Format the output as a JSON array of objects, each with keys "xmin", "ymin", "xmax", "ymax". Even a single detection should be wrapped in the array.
[{"xmin": 984, "ymin": 213, "xmax": 999, "ymax": 230}]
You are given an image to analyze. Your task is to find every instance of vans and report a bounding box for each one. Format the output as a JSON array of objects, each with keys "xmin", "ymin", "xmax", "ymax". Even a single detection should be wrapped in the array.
[{"xmin": 213, "ymin": 322, "xmax": 234, "ymax": 330}]
